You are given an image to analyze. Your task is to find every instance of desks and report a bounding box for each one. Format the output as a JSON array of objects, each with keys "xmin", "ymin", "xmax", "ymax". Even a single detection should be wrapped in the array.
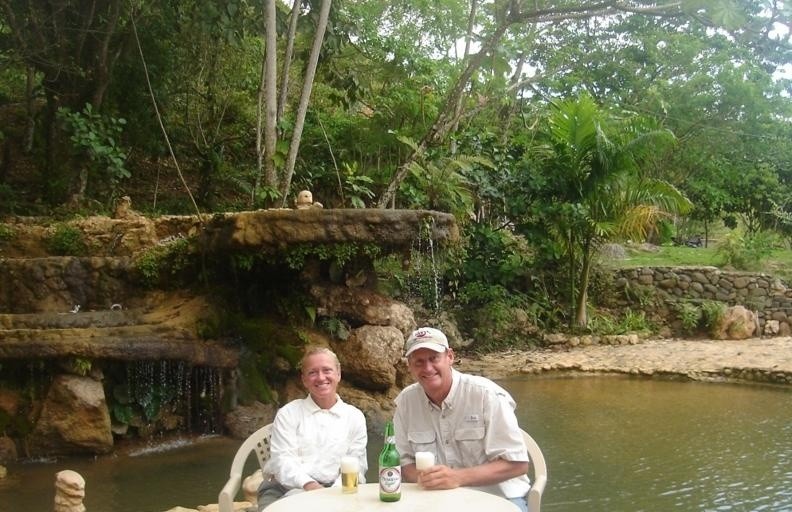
[{"xmin": 259, "ymin": 481, "xmax": 525, "ymax": 512}]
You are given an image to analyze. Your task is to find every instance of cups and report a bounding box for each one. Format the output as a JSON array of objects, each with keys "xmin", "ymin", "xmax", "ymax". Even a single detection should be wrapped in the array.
[
  {"xmin": 341, "ymin": 457, "xmax": 360, "ymax": 495},
  {"xmin": 414, "ymin": 446, "xmax": 436, "ymax": 489}
]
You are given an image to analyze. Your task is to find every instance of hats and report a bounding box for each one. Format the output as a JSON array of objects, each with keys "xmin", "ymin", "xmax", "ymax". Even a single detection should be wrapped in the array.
[{"xmin": 404, "ymin": 327, "xmax": 449, "ymax": 358}]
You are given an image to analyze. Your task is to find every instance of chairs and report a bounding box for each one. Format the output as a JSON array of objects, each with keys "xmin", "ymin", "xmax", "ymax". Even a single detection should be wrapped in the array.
[
  {"xmin": 515, "ymin": 427, "xmax": 552, "ymax": 512},
  {"xmin": 218, "ymin": 420, "xmax": 276, "ymax": 510}
]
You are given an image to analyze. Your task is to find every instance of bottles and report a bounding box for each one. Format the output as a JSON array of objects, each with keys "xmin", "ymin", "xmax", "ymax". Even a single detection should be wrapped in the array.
[{"xmin": 377, "ymin": 422, "xmax": 403, "ymax": 502}]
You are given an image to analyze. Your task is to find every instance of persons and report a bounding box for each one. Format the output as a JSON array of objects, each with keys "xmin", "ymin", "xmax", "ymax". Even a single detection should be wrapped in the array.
[
  {"xmin": 393, "ymin": 327, "xmax": 529, "ymax": 512},
  {"xmin": 256, "ymin": 348, "xmax": 368, "ymax": 512}
]
